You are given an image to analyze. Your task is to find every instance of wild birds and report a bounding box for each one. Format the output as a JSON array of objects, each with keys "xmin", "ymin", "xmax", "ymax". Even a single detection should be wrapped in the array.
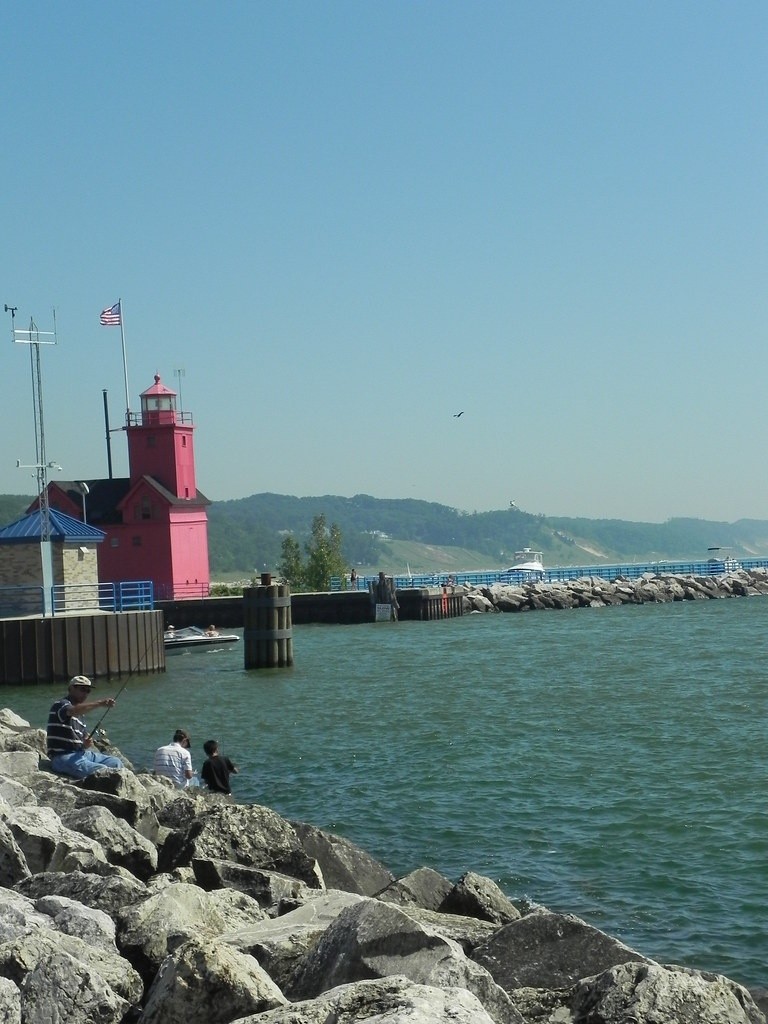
[{"xmin": 453, "ymin": 411, "xmax": 464, "ymax": 418}]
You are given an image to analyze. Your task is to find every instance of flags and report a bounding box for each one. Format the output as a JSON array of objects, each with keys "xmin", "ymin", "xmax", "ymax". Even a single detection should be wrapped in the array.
[{"xmin": 100, "ymin": 303, "xmax": 120, "ymax": 326}]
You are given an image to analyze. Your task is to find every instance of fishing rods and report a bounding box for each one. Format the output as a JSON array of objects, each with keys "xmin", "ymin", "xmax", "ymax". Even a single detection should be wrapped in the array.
[{"xmin": 87, "ymin": 597, "xmax": 184, "ymax": 740}]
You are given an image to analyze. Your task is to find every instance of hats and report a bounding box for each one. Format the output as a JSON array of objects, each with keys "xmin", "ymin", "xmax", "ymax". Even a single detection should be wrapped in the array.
[
  {"xmin": 68, "ymin": 676, "xmax": 96, "ymax": 688},
  {"xmin": 176, "ymin": 729, "xmax": 191, "ymax": 748}
]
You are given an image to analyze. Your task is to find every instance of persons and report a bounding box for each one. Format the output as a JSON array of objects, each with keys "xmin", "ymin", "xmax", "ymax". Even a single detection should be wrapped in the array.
[
  {"xmin": 207, "ymin": 625, "xmax": 219, "ymax": 637},
  {"xmin": 350, "ymin": 569, "xmax": 358, "ymax": 591},
  {"xmin": 200, "ymin": 740, "xmax": 238, "ymax": 795},
  {"xmin": 46, "ymin": 675, "xmax": 123, "ymax": 779},
  {"xmin": 449, "ymin": 575, "xmax": 453, "ymax": 585},
  {"xmin": 166, "ymin": 625, "xmax": 174, "ymax": 636},
  {"xmin": 153, "ymin": 729, "xmax": 199, "ymax": 789}
]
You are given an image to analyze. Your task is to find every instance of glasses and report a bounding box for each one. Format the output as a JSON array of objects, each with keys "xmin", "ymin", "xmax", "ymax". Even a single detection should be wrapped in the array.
[{"xmin": 75, "ymin": 686, "xmax": 91, "ymax": 693}]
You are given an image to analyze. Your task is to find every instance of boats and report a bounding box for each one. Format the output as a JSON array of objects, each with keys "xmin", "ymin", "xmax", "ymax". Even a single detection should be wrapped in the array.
[
  {"xmin": 706, "ymin": 547, "xmax": 742, "ymax": 573},
  {"xmin": 633, "ymin": 563, "xmax": 639, "ymax": 566},
  {"xmin": 163, "ymin": 626, "xmax": 240, "ymax": 657},
  {"xmin": 649, "ymin": 560, "xmax": 667, "ymax": 565},
  {"xmin": 508, "ymin": 548, "xmax": 545, "ymax": 583}
]
